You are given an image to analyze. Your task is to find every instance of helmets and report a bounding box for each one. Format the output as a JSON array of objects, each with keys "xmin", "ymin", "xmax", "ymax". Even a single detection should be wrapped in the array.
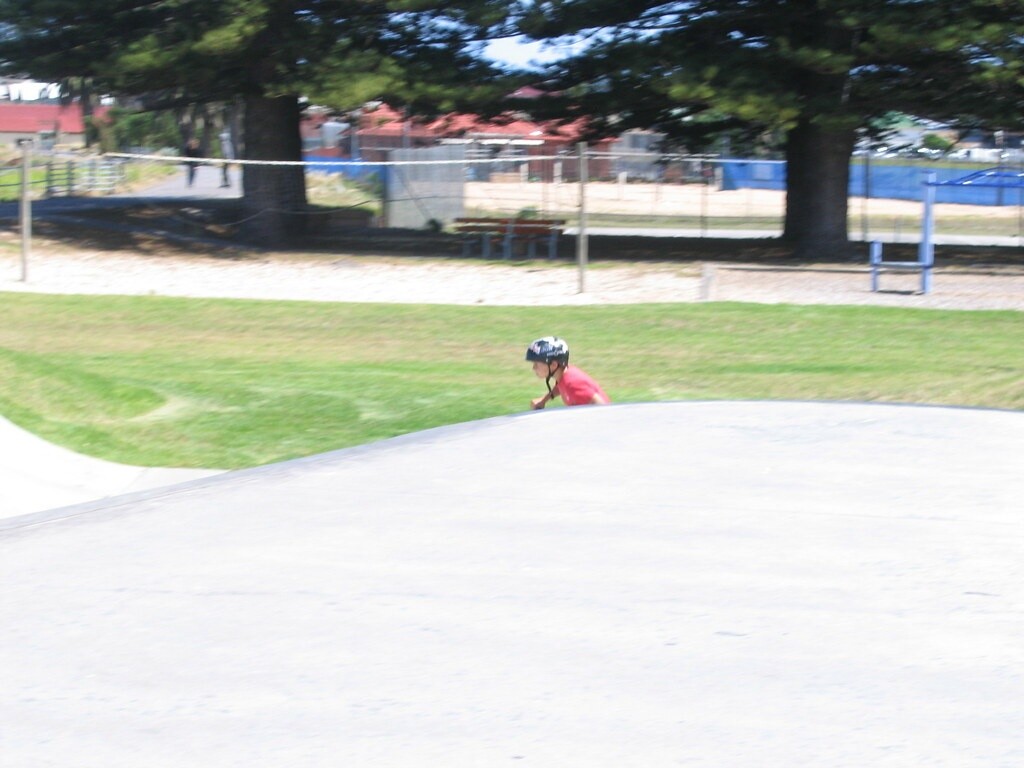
[{"xmin": 525, "ymin": 336, "xmax": 569, "ymax": 368}]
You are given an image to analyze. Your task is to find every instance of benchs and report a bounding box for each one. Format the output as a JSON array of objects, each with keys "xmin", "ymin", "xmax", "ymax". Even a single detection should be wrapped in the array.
[{"xmin": 451, "ymin": 216, "xmax": 566, "ymax": 263}]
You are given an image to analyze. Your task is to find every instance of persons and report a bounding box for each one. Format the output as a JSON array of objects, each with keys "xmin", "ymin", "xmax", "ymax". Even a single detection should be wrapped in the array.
[
  {"xmin": 219, "ymin": 134, "xmax": 233, "ymax": 187},
  {"xmin": 185, "ymin": 139, "xmax": 202, "ymax": 186},
  {"xmin": 525, "ymin": 337, "xmax": 610, "ymax": 410}
]
